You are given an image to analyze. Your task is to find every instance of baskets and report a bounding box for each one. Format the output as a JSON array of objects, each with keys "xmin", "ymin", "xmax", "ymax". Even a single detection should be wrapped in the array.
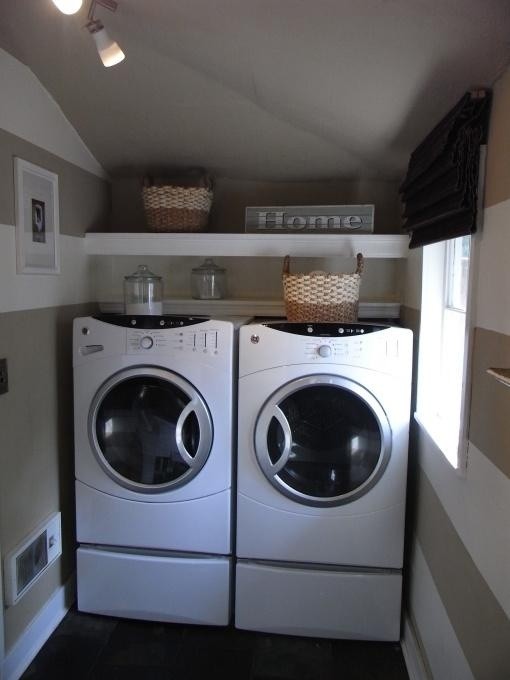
[
  {"xmin": 137, "ymin": 167, "xmax": 215, "ymax": 233},
  {"xmin": 281, "ymin": 252, "xmax": 365, "ymax": 323}
]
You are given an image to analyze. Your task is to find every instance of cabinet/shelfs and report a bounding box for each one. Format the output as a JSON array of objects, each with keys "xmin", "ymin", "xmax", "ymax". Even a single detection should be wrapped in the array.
[{"xmin": 84, "ymin": 231, "xmax": 412, "ymax": 321}]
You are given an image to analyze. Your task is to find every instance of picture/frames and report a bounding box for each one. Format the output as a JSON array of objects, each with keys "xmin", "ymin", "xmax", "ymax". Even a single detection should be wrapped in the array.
[{"xmin": 14, "ymin": 157, "xmax": 63, "ymax": 276}]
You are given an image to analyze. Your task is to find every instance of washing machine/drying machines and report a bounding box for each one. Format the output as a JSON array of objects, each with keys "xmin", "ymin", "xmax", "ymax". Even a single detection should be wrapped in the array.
[
  {"xmin": 71, "ymin": 316, "xmax": 235, "ymax": 626},
  {"xmin": 236, "ymin": 318, "xmax": 414, "ymax": 642}
]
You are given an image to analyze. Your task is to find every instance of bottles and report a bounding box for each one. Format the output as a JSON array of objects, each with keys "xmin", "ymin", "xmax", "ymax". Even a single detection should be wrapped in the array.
[
  {"xmin": 191, "ymin": 256, "xmax": 227, "ymax": 300},
  {"xmin": 123, "ymin": 266, "xmax": 164, "ymax": 319}
]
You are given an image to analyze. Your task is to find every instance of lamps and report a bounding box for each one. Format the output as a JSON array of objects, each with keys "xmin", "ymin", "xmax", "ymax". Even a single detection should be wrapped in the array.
[{"xmin": 85, "ymin": 0, "xmax": 126, "ymax": 68}]
